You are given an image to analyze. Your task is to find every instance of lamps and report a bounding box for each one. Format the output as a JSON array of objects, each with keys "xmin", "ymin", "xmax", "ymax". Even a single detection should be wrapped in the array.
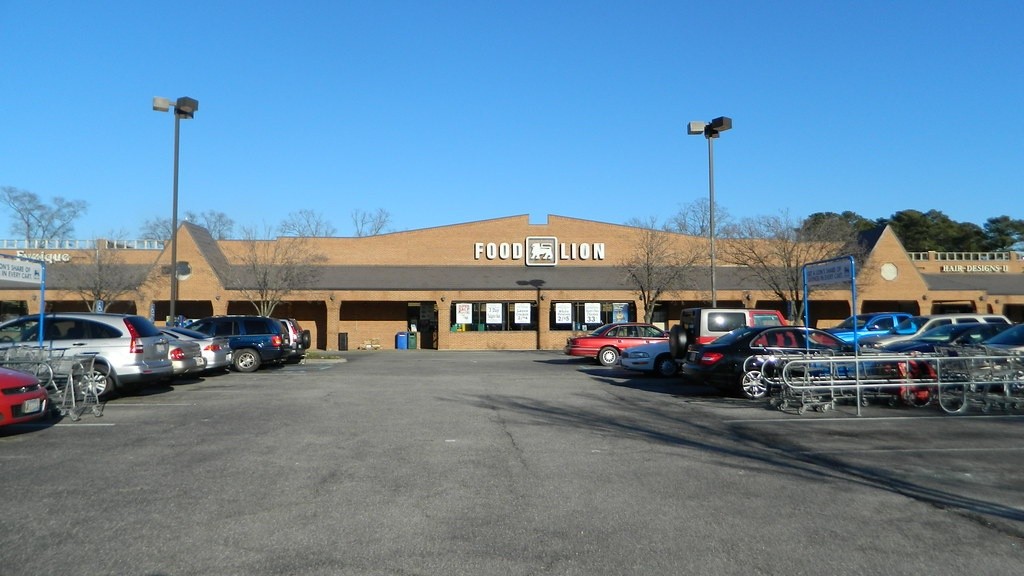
[
  {"xmin": 640, "ymin": 294, "xmax": 643, "ymax": 299},
  {"xmin": 441, "ymin": 296, "xmax": 444, "ymax": 301},
  {"xmin": 541, "ymin": 293, "xmax": 544, "ymax": 300},
  {"xmin": 331, "ymin": 294, "xmax": 335, "ymax": 299},
  {"xmin": 748, "ymin": 294, "xmax": 752, "ymax": 300}
]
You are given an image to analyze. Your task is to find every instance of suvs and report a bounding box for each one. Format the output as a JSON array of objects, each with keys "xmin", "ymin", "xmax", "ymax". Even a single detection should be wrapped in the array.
[
  {"xmin": 667, "ymin": 308, "xmax": 791, "ymax": 373},
  {"xmin": 0, "ymin": 313, "xmax": 174, "ymax": 402},
  {"xmin": 180, "ymin": 315, "xmax": 293, "ymax": 372},
  {"xmin": 279, "ymin": 318, "xmax": 311, "ymax": 365},
  {"xmin": 858, "ymin": 312, "xmax": 1014, "ymax": 352}
]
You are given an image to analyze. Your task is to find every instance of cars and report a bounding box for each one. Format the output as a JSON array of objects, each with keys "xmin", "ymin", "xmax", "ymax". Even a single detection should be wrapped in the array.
[
  {"xmin": 0, "ymin": 368, "xmax": 50, "ymax": 434},
  {"xmin": 881, "ymin": 322, "xmax": 1013, "ymax": 353},
  {"xmin": 975, "ymin": 322, "xmax": 1024, "ymax": 353},
  {"xmin": 563, "ymin": 323, "xmax": 672, "ymax": 367},
  {"xmin": 619, "ymin": 340, "xmax": 676, "ymax": 378},
  {"xmin": 813, "ymin": 312, "xmax": 920, "ymax": 349},
  {"xmin": 680, "ymin": 325, "xmax": 865, "ymax": 400},
  {"xmin": 156, "ymin": 327, "xmax": 233, "ymax": 372},
  {"xmin": 150, "ymin": 328, "xmax": 207, "ymax": 378}
]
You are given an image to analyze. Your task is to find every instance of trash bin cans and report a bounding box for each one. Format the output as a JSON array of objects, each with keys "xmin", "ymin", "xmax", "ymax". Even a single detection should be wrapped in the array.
[
  {"xmin": 408, "ymin": 332, "xmax": 417, "ymax": 349},
  {"xmin": 339, "ymin": 333, "xmax": 348, "ymax": 350},
  {"xmin": 397, "ymin": 332, "xmax": 407, "ymax": 349}
]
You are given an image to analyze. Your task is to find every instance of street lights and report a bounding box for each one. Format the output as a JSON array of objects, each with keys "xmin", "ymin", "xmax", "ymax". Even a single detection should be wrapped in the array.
[
  {"xmin": 688, "ymin": 116, "xmax": 733, "ymax": 310},
  {"xmin": 153, "ymin": 94, "xmax": 200, "ymax": 324}
]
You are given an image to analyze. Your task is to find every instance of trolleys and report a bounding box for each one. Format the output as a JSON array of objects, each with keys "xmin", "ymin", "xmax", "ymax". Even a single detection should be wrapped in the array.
[{"xmin": 3, "ymin": 343, "xmax": 106, "ymax": 421}]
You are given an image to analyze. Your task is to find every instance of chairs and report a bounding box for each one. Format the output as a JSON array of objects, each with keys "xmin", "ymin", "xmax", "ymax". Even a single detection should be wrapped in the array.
[
  {"xmin": 47, "ymin": 325, "xmax": 62, "ymax": 337},
  {"xmin": 65, "ymin": 328, "xmax": 81, "ymax": 338}
]
